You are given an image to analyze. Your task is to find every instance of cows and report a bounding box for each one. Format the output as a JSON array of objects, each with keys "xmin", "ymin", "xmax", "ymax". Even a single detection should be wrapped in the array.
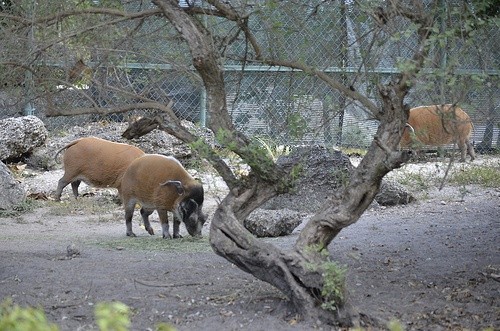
[
  {"xmin": 119, "ymin": 154, "xmax": 209, "ymax": 240},
  {"xmin": 52, "ymin": 135, "xmax": 147, "ymax": 206},
  {"xmin": 400, "ymin": 104, "xmax": 477, "ymax": 164}
]
